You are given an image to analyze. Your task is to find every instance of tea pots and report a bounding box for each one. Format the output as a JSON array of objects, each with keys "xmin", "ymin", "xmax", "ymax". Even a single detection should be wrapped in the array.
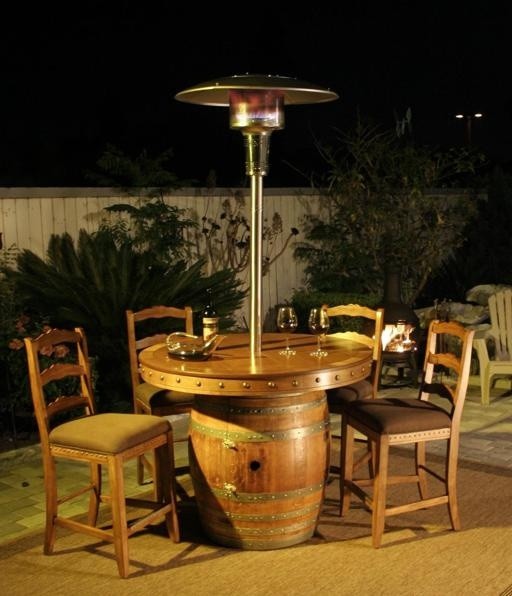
[{"xmin": 162, "ymin": 331, "xmax": 230, "ymax": 362}]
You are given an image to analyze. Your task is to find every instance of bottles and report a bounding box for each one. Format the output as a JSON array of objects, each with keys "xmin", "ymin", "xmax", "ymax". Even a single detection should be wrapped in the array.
[{"xmin": 202, "ymin": 304, "xmax": 218, "ymax": 346}]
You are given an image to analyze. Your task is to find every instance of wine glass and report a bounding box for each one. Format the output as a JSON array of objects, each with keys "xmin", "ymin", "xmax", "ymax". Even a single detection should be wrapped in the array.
[
  {"xmin": 307, "ymin": 308, "xmax": 329, "ymax": 358},
  {"xmin": 276, "ymin": 307, "xmax": 299, "ymax": 357}
]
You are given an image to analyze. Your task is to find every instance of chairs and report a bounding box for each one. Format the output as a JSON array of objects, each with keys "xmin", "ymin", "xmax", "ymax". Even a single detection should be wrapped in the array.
[
  {"xmin": 24, "ymin": 328, "xmax": 180, "ymax": 579},
  {"xmin": 320, "ymin": 305, "xmax": 384, "ymax": 479},
  {"xmin": 340, "ymin": 320, "xmax": 475, "ymax": 548},
  {"xmin": 126, "ymin": 307, "xmax": 194, "ymax": 503},
  {"xmin": 473, "ymin": 289, "xmax": 512, "ymax": 405}
]
